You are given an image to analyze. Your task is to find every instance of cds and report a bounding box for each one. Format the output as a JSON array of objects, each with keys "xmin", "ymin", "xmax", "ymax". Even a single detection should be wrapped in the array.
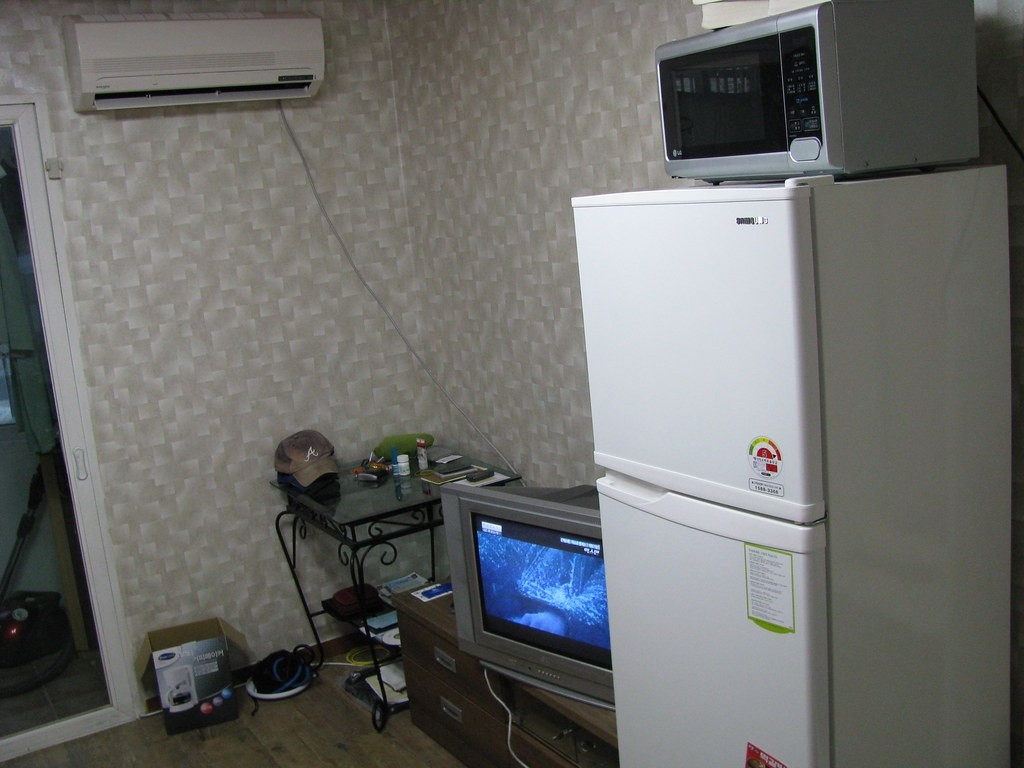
[{"xmin": 383, "ymin": 628, "xmax": 401, "ymax": 645}]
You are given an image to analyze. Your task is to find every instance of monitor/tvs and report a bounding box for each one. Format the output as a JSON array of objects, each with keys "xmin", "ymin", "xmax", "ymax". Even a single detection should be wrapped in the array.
[{"xmin": 439, "ymin": 481, "xmax": 616, "ymax": 712}]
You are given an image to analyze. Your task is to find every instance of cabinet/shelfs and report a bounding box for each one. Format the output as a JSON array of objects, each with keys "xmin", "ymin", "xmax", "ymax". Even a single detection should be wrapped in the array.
[{"xmin": 390, "ymin": 594, "xmax": 616, "ymax": 768}]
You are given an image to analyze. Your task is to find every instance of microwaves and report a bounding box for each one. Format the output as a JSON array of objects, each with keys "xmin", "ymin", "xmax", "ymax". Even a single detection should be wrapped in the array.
[{"xmin": 654, "ymin": 0, "xmax": 981, "ymax": 186}]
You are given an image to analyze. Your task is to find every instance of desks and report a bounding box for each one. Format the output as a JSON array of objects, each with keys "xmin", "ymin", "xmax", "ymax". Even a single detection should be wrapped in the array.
[{"xmin": 276, "ymin": 446, "xmax": 522, "ymax": 733}]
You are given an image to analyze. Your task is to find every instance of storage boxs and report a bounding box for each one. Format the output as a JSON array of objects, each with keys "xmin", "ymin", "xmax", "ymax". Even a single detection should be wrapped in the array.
[{"xmin": 137, "ymin": 619, "xmax": 246, "ymax": 736}]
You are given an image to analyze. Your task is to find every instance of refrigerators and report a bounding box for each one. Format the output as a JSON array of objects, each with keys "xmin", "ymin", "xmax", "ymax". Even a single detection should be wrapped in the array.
[{"xmin": 571, "ymin": 163, "xmax": 1017, "ymax": 768}]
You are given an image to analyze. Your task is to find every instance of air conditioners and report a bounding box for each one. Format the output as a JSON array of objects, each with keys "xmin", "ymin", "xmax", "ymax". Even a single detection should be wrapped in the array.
[{"xmin": 62, "ymin": 13, "xmax": 326, "ymax": 114}]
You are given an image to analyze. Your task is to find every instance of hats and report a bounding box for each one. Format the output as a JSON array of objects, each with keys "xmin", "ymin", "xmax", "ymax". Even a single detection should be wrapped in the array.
[{"xmin": 275, "ymin": 432, "xmax": 338, "ymax": 490}]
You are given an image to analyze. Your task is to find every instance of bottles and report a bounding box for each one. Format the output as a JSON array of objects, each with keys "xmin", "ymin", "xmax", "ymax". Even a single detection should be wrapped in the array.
[
  {"xmin": 416, "ymin": 439, "xmax": 428, "ymax": 470},
  {"xmin": 397, "ymin": 455, "xmax": 410, "ymax": 475}
]
